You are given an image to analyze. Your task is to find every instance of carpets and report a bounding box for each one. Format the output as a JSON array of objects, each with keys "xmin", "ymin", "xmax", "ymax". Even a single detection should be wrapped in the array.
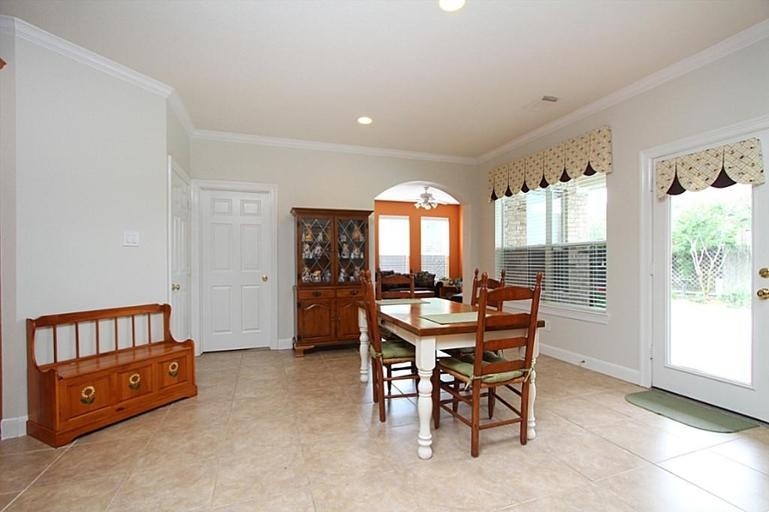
[{"xmin": 624, "ymin": 388, "xmax": 761, "ymax": 434}]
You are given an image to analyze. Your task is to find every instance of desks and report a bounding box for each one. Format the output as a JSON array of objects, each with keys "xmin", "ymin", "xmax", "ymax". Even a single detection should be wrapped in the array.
[{"xmin": 354, "ymin": 297, "xmax": 545, "ymax": 459}]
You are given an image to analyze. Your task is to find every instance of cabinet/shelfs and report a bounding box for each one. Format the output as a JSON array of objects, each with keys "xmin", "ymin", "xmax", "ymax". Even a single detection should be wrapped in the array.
[
  {"xmin": 290, "ymin": 207, "xmax": 375, "ymax": 358},
  {"xmin": 25, "ymin": 304, "xmax": 198, "ymax": 449}
]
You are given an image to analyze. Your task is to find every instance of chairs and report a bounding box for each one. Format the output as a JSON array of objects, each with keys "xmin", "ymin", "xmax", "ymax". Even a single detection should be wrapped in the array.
[{"xmin": 360, "ymin": 269, "xmax": 543, "ymax": 457}]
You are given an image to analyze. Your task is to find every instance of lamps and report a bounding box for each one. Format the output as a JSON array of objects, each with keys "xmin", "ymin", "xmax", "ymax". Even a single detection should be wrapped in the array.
[{"xmin": 414, "ymin": 187, "xmax": 439, "ymax": 210}]
